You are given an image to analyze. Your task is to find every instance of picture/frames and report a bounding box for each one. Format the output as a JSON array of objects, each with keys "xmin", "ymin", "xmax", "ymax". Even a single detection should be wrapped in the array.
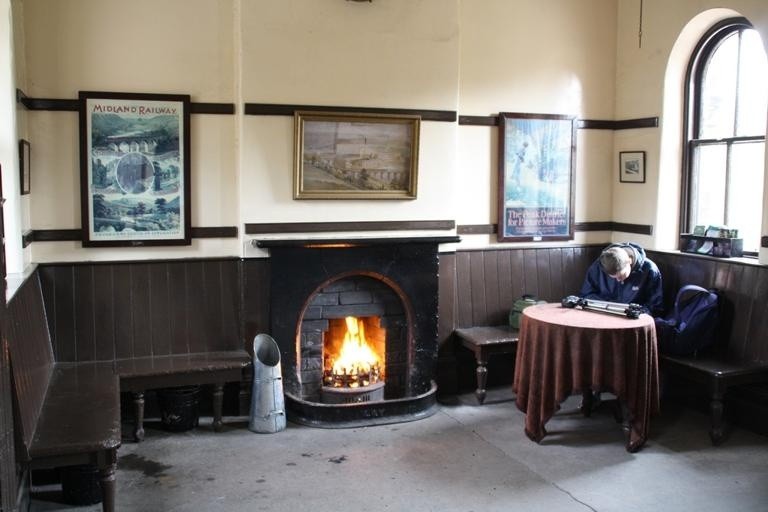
[
  {"xmin": 78, "ymin": 91, "xmax": 191, "ymax": 249},
  {"xmin": 19, "ymin": 138, "xmax": 31, "ymax": 196},
  {"xmin": 293, "ymin": 108, "xmax": 421, "ymax": 200},
  {"xmin": 497, "ymin": 112, "xmax": 578, "ymax": 242},
  {"xmin": 619, "ymin": 150, "xmax": 646, "ymax": 183}
]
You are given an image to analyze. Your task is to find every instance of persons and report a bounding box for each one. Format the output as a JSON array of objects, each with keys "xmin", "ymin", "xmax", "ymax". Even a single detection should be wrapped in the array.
[{"xmin": 577, "ymin": 241, "xmax": 664, "ymax": 415}]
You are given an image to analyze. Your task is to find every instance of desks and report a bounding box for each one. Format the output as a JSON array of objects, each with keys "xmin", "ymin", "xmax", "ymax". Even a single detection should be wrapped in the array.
[{"xmin": 513, "ymin": 303, "xmax": 662, "ymax": 454}]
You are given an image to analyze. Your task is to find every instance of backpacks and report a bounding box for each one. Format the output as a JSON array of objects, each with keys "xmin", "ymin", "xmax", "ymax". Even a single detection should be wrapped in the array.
[{"xmin": 656, "ymin": 284, "xmax": 727, "ymax": 359}]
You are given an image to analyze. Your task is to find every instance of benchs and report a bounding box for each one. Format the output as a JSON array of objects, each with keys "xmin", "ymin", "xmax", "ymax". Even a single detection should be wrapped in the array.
[
  {"xmin": 6, "ymin": 255, "xmax": 253, "ymax": 512},
  {"xmin": 456, "ymin": 242, "xmax": 768, "ymax": 446}
]
